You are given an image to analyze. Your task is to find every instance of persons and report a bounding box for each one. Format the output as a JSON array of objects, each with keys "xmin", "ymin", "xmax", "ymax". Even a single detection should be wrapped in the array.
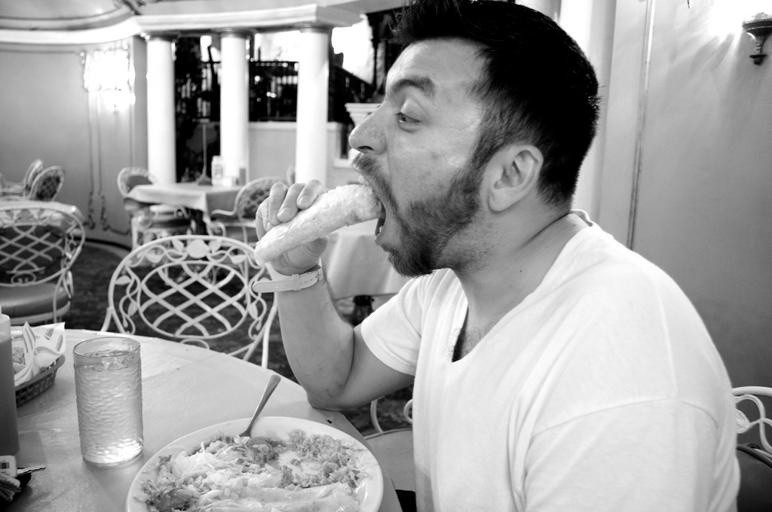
[{"xmin": 250, "ymin": 1, "xmax": 744, "ymax": 510}]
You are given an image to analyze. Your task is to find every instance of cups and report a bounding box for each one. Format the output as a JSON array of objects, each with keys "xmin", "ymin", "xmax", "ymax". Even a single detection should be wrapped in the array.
[
  {"xmin": 74, "ymin": 334, "xmax": 146, "ymax": 469},
  {"xmin": 211, "ymin": 156, "xmax": 225, "ymax": 187}
]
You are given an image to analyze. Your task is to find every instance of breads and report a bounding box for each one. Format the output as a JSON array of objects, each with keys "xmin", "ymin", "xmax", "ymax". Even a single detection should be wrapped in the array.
[{"xmin": 253, "ymin": 185, "xmax": 382, "ymax": 267}]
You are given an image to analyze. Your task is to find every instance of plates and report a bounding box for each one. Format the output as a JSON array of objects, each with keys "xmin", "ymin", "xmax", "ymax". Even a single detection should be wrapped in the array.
[{"xmin": 124, "ymin": 416, "xmax": 386, "ymax": 512}]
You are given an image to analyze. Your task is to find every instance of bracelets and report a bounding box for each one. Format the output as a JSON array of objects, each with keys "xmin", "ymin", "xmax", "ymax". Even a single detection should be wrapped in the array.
[{"xmin": 250, "ymin": 266, "xmax": 324, "ymax": 295}]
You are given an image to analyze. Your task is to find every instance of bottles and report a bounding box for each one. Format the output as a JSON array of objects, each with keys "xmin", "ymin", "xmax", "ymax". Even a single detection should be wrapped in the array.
[{"xmin": 0, "ymin": 311, "xmax": 21, "ymax": 459}]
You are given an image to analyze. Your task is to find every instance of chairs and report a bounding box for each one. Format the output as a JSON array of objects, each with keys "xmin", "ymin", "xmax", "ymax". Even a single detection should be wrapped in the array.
[
  {"xmin": 105, "ymin": 231, "xmax": 285, "ymax": 366},
  {"xmin": 207, "ymin": 176, "xmax": 292, "ymax": 294},
  {"xmin": 2, "ymin": 158, "xmax": 68, "ymax": 203},
  {"xmin": 0, "ymin": 203, "xmax": 88, "ymax": 333},
  {"xmin": 726, "ymin": 383, "xmax": 771, "ymax": 512},
  {"xmin": 114, "ymin": 164, "xmax": 183, "ymax": 279}
]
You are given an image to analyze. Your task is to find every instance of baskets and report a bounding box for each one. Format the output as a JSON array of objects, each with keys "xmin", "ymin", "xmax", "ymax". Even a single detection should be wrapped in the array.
[{"xmin": 14, "ymin": 353, "xmax": 65, "ymax": 407}]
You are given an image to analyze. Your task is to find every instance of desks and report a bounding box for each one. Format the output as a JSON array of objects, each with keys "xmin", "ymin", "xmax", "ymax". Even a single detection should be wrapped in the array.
[
  {"xmin": 2, "ymin": 325, "xmax": 400, "ymax": 511},
  {"xmin": 124, "ymin": 180, "xmax": 256, "ymax": 232}
]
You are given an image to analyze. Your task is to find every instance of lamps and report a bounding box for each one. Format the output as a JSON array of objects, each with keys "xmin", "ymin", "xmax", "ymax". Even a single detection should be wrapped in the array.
[{"xmin": 739, "ymin": 11, "xmax": 772, "ymax": 67}]
